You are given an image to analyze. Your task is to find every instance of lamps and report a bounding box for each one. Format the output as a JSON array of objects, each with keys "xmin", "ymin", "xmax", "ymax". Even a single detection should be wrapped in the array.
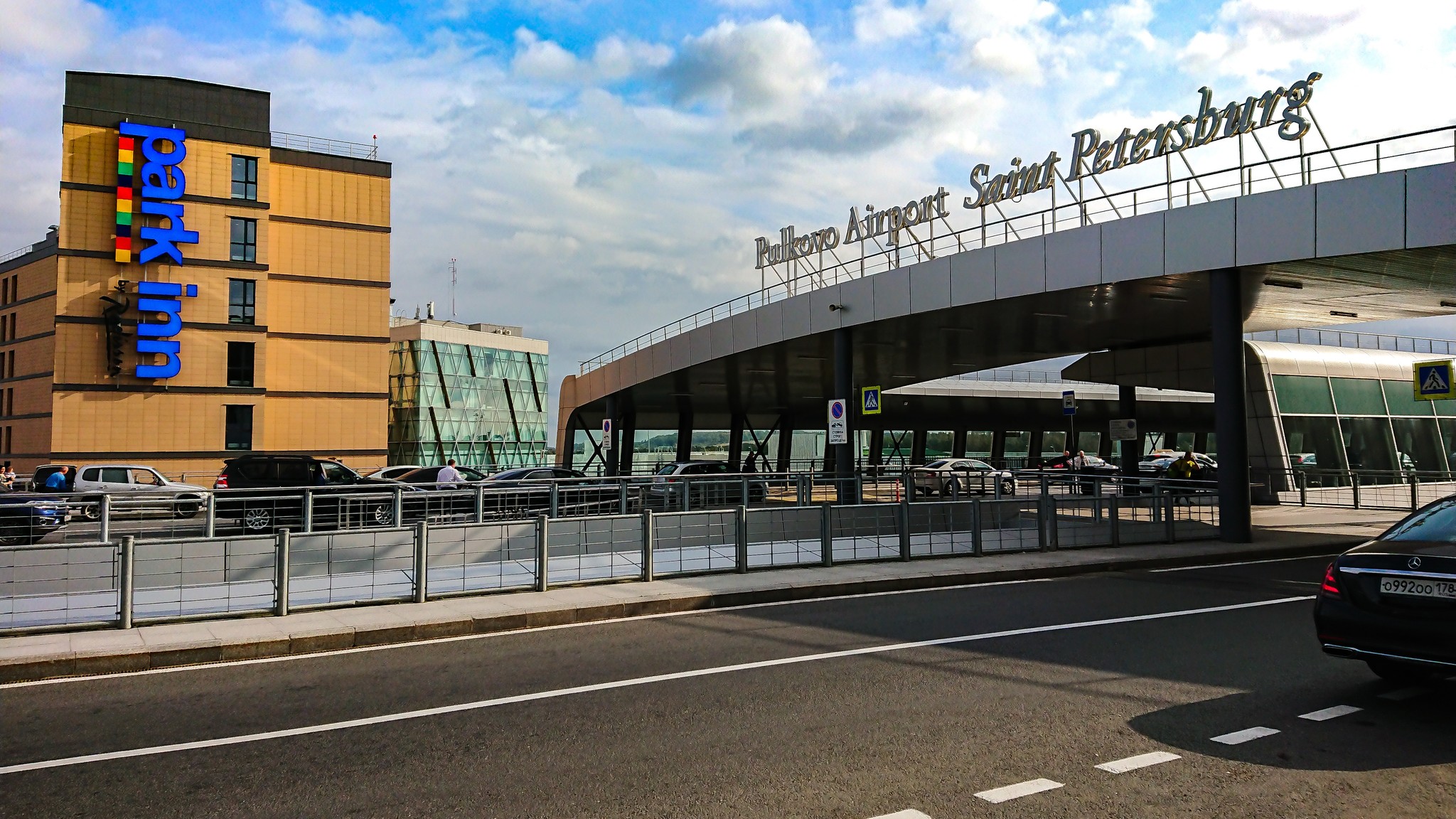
[{"xmin": 828, "ymin": 304, "xmax": 844, "ymax": 312}]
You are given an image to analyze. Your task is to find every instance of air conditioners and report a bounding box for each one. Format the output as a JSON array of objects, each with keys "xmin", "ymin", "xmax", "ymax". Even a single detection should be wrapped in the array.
[
  {"xmin": 497, "ymin": 329, "xmax": 502, "ymax": 334},
  {"xmin": 505, "ymin": 330, "xmax": 512, "ymax": 335}
]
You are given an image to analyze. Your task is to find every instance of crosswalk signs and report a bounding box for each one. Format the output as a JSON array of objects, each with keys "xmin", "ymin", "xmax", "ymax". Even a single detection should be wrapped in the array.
[
  {"xmin": 858, "ymin": 386, "xmax": 884, "ymax": 417},
  {"xmin": 1410, "ymin": 357, "xmax": 1455, "ymax": 403}
]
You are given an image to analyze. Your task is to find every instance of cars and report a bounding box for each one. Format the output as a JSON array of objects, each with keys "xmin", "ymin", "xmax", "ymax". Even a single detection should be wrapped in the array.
[
  {"xmin": 0, "ymin": 481, "xmax": 71, "ymax": 547},
  {"xmin": 455, "ymin": 467, "xmax": 633, "ymax": 522},
  {"xmin": 391, "ymin": 465, "xmax": 490, "ymax": 514},
  {"xmin": 1037, "ymin": 455, "xmax": 1122, "ymax": 484},
  {"xmin": 910, "ymin": 458, "xmax": 1018, "ymax": 496},
  {"xmin": 1314, "ymin": 490, "xmax": 1456, "ymax": 682},
  {"xmin": 361, "ymin": 465, "xmax": 424, "ymax": 479},
  {"xmin": 1398, "ymin": 451, "xmax": 1421, "ymax": 483}
]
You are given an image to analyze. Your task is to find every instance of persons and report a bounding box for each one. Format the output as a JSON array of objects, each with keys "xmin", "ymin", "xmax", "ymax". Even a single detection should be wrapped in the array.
[
  {"xmin": 741, "ymin": 451, "xmax": 759, "ymax": 473},
  {"xmin": 325, "ymin": 457, "xmax": 338, "ymax": 479},
  {"xmin": 0, "ymin": 464, "xmax": 16, "ymax": 490},
  {"xmin": 1062, "ymin": 451, "xmax": 1073, "ymax": 486},
  {"xmin": 44, "ymin": 466, "xmax": 69, "ymax": 493},
  {"xmin": 1074, "ymin": 450, "xmax": 1089, "ymax": 474},
  {"xmin": 436, "ymin": 459, "xmax": 469, "ymax": 525},
  {"xmin": 1174, "ymin": 451, "xmax": 1200, "ymax": 507}
]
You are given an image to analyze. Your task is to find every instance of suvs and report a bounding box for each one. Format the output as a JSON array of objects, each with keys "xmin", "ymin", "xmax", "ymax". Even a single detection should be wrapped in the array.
[
  {"xmin": 30, "ymin": 463, "xmax": 209, "ymax": 523},
  {"xmin": 1289, "ymin": 453, "xmax": 1318, "ymax": 471},
  {"xmin": 650, "ymin": 459, "xmax": 770, "ymax": 508},
  {"xmin": 213, "ymin": 455, "xmax": 416, "ymax": 535},
  {"xmin": 1139, "ymin": 451, "xmax": 1218, "ymax": 494}
]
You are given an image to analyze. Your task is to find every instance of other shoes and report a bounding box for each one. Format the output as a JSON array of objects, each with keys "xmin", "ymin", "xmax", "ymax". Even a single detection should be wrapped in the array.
[
  {"xmin": 1188, "ymin": 501, "xmax": 1195, "ymax": 505},
  {"xmin": 1174, "ymin": 502, "xmax": 1182, "ymax": 507}
]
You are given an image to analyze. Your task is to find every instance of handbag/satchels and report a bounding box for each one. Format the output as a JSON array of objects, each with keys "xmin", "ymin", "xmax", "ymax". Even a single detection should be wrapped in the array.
[{"xmin": 1168, "ymin": 476, "xmax": 1178, "ymax": 486}]
